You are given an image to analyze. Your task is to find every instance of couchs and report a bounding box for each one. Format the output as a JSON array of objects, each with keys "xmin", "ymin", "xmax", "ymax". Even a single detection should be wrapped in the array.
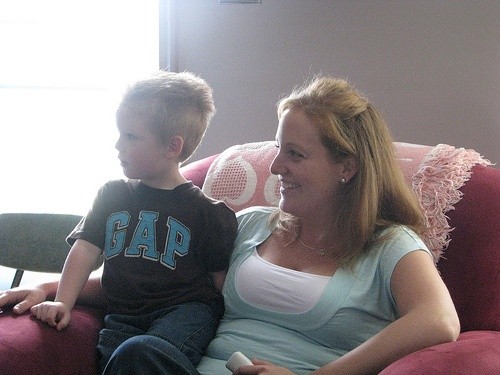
[{"xmin": 0, "ymin": 139, "xmax": 500, "ymax": 374}]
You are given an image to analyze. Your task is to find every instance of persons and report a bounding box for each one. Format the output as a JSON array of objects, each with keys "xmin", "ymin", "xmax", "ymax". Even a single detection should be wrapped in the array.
[
  {"xmin": 30, "ymin": 71, "xmax": 239, "ymax": 375},
  {"xmin": 1, "ymin": 78, "xmax": 461, "ymax": 375}
]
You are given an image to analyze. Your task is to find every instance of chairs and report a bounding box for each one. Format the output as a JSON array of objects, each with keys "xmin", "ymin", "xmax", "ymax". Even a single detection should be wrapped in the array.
[{"xmin": 0, "ymin": 213, "xmax": 84, "ymax": 289}]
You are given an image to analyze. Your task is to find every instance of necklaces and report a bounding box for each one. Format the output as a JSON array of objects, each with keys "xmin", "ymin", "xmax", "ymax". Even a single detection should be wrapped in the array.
[{"xmin": 294, "ymin": 229, "xmax": 344, "ymax": 254}]
